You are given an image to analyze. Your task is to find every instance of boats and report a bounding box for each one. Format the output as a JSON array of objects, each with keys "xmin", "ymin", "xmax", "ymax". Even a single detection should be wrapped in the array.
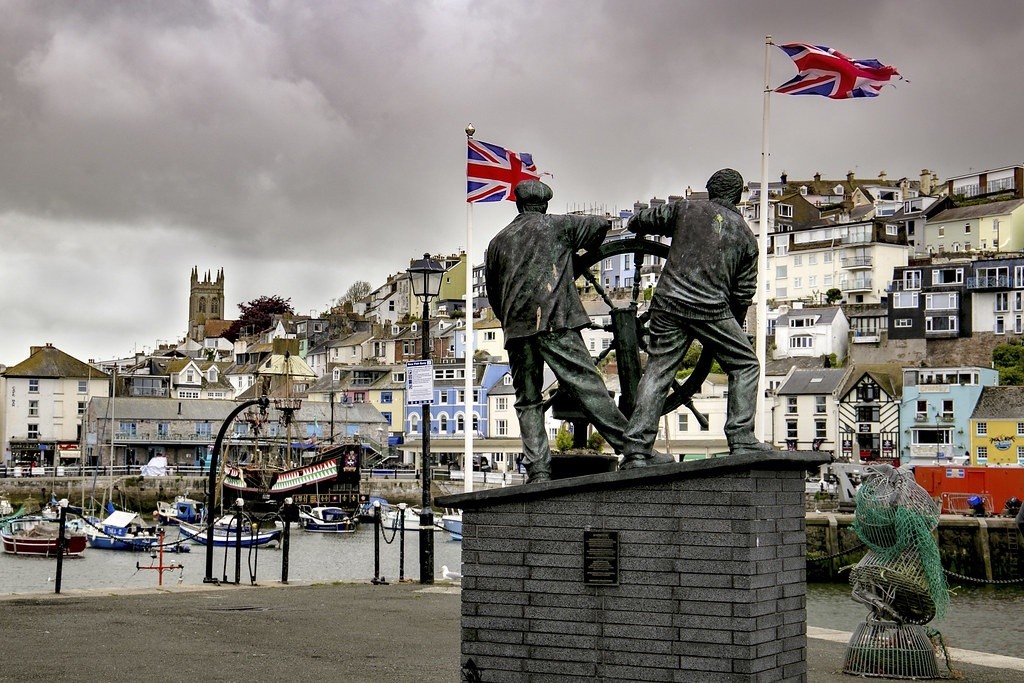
[
  {"xmin": 180, "ymin": 515, "xmax": 283, "ymax": 550},
  {"xmin": 357, "ymin": 497, "xmax": 389, "ymax": 524},
  {"xmin": 380, "ymin": 503, "xmax": 444, "ymax": 531},
  {"xmin": 2, "ymin": 495, "xmax": 87, "ymax": 556},
  {"xmin": 442, "ymin": 507, "xmax": 462, "ymax": 541},
  {"xmin": 299, "ymin": 505, "xmax": 357, "ymax": 533},
  {"xmin": 155, "ymin": 495, "xmax": 208, "ymax": 525}
]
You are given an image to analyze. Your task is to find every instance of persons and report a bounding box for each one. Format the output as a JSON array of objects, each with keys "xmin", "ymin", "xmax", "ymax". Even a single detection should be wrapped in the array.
[
  {"xmin": 15, "ymin": 523, "xmax": 23, "ymax": 532},
  {"xmin": 619, "ymin": 168, "xmax": 776, "ymax": 470},
  {"xmin": 200, "ymin": 457, "xmax": 206, "ymax": 476},
  {"xmin": 486, "ymin": 179, "xmax": 676, "ymax": 484}
]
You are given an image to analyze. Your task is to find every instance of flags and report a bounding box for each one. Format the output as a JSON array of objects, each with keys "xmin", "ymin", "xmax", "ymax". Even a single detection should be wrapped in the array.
[
  {"xmin": 467, "ymin": 139, "xmax": 553, "ymax": 203},
  {"xmin": 773, "ymin": 43, "xmax": 903, "ymax": 100}
]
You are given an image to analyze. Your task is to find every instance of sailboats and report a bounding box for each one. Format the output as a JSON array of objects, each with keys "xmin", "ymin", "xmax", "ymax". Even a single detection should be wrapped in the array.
[
  {"xmin": 217, "ymin": 325, "xmax": 364, "ymax": 522},
  {"xmin": 42, "ymin": 360, "xmax": 160, "ymax": 551}
]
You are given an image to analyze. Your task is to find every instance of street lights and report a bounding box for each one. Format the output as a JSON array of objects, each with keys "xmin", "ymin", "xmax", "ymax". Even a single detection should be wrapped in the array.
[{"xmin": 404, "ymin": 252, "xmax": 448, "ymax": 584}]
